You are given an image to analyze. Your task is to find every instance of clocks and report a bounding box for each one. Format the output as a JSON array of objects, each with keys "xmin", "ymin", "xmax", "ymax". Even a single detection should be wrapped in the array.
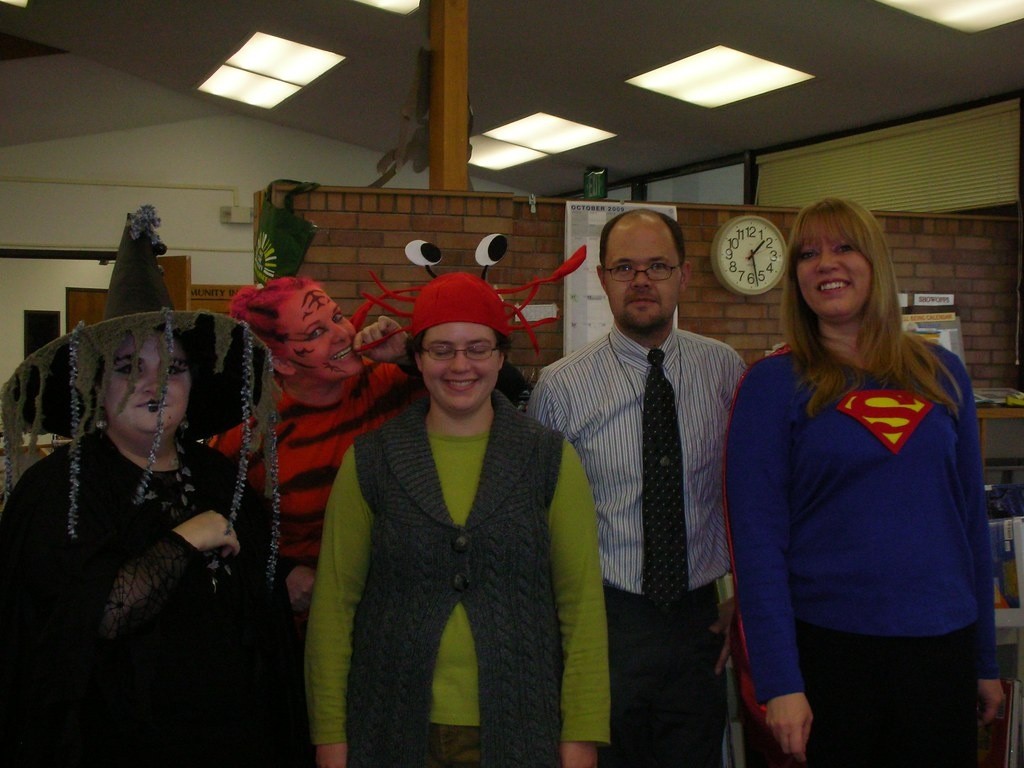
[{"xmin": 710, "ymin": 215, "xmax": 787, "ymax": 295}]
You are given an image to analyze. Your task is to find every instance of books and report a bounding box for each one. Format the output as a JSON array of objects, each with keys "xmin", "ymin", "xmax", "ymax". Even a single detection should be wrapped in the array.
[
  {"xmin": 975, "ymin": 676, "xmax": 1024, "ymax": 768},
  {"xmin": 987, "ymin": 515, "xmax": 1021, "ymax": 609}
]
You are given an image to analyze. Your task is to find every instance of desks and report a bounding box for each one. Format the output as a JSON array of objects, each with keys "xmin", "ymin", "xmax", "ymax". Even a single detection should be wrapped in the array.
[{"xmin": 973, "ymin": 408, "xmax": 1024, "ymax": 470}]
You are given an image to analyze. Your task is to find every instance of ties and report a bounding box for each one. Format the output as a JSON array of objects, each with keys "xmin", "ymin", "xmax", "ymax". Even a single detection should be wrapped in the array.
[{"xmin": 641, "ymin": 349, "xmax": 689, "ymax": 610}]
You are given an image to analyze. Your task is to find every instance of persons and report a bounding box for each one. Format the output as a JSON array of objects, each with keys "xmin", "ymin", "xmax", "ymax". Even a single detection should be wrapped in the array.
[
  {"xmin": 229, "ymin": 276, "xmax": 535, "ymax": 638},
  {"xmin": 522, "ymin": 209, "xmax": 749, "ymax": 768},
  {"xmin": 721, "ymin": 197, "xmax": 1006, "ymax": 768},
  {"xmin": 0, "ymin": 204, "xmax": 317, "ymax": 767},
  {"xmin": 304, "ymin": 270, "xmax": 612, "ymax": 768}
]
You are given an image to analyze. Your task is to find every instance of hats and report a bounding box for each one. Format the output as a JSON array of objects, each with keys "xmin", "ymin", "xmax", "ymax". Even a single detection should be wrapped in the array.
[
  {"xmin": 0, "ymin": 212, "xmax": 272, "ymax": 439},
  {"xmin": 347, "ymin": 233, "xmax": 587, "ymax": 358}
]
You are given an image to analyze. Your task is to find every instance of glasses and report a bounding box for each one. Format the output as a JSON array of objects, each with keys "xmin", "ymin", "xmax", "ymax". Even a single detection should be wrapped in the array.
[
  {"xmin": 604, "ymin": 261, "xmax": 682, "ymax": 282},
  {"xmin": 419, "ymin": 345, "xmax": 498, "ymax": 360}
]
